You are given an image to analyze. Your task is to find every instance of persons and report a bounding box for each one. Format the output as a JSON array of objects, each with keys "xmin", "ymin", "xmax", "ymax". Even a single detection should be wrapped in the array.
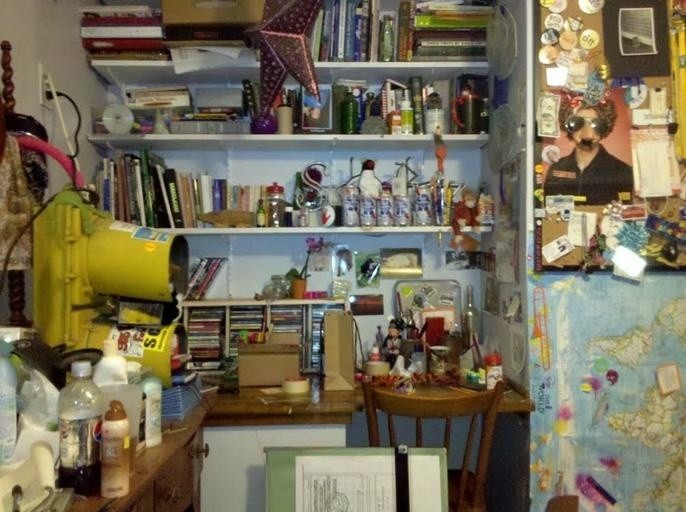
[{"xmin": 543, "ymin": 94, "xmax": 633, "ymax": 205}]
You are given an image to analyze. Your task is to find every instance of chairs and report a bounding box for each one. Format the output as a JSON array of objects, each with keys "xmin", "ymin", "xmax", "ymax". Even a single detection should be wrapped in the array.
[{"xmin": 362, "ymin": 379, "xmax": 507, "ymax": 512}]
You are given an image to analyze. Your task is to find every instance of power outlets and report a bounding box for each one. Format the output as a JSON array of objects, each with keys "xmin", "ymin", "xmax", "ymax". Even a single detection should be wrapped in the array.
[{"xmin": 37, "ymin": 64, "xmax": 52, "ymax": 111}]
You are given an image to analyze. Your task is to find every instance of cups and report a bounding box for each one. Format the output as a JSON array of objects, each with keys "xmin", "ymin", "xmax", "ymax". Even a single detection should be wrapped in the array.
[
  {"xmin": 452, "ymin": 95, "xmax": 480, "ymax": 134},
  {"xmin": 291, "ymin": 278, "xmax": 307, "ymax": 299}
]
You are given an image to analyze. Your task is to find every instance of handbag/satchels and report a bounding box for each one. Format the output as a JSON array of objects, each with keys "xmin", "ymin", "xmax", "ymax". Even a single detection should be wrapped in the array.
[{"xmin": 323, "ymin": 311, "xmax": 354, "ymax": 390}]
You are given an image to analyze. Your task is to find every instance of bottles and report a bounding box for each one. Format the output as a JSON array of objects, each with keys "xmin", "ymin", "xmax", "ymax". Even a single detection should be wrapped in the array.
[
  {"xmin": 257, "ymin": 172, "xmax": 306, "ymax": 227},
  {"xmin": 58, "ymin": 360, "xmax": 131, "ymax": 500},
  {"xmin": 340, "ymin": 91, "xmax": 358, "ymax": 134},
  {"xmin": 464, "ymin": 286, "xmax": 480, "ymax": 346},
  {"xmin": 486, "ymin": 354, "xmax": 504, "ymax": 392},
  {"xmin": 0, "ymin": 340, "xmax": 17, "ymax": 463},
  {"xmin": 380, "ymin": 15, "xmax": 395, "ymax": 62}
]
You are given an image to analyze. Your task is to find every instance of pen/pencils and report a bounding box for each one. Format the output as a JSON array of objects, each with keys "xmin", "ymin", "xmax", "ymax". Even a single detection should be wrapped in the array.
[
  {"xmin": 393, "ymin": 377, "xmax": 415, "ymax": 394},
  {"xmin": 588, "ymin": 476, "xmax": 617, "ymax": 505}
]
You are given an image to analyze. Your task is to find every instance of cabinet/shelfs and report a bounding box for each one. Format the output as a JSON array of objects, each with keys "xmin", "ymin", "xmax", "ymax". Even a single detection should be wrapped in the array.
[{"xmin": 79, "ymin": 58, "xmax": 491, "ymax": 234}]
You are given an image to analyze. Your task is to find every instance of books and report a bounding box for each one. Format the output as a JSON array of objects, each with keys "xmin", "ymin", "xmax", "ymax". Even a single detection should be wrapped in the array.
[
  {"xmin": 311, "ymin": 0, "xmax": 380, "ymax": 62},
  {"xmin": 396, "ymin": 0, "xmax": 495, "ymax": 61},
  {"xmin": 80, "ymin": 5, "xmax": 170, "ymax": 60},
  {"xmin": 187, "ymin": 306, "xmax": 344, "ymax": 370},
  {"xmin": 96, "ymin": 147, "xmax": 269, "ymax": 229}
]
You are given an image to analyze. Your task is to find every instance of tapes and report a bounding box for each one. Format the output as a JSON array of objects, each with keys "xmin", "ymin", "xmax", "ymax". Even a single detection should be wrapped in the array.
[{"xmin": 281, "ymin": 377, "xmax": 310, "ymax": 393}]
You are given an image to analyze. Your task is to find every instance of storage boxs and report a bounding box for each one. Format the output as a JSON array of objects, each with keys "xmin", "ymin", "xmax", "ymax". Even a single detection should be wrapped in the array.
[{"xmin": 239, "ymin": 331, "xmax": 301, "ymax": 387}]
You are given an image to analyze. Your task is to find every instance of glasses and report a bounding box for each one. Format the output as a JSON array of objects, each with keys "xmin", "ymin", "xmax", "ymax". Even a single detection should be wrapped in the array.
[{"xmin": 565, "ymin": 117, "xmax": 607, "ymax": 134}]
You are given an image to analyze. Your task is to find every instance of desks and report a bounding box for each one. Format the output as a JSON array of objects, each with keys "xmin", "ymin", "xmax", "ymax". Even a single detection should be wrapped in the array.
[{"xmin": 0, "ymin": 402, "xmax": 210, "ymax": 512}]
